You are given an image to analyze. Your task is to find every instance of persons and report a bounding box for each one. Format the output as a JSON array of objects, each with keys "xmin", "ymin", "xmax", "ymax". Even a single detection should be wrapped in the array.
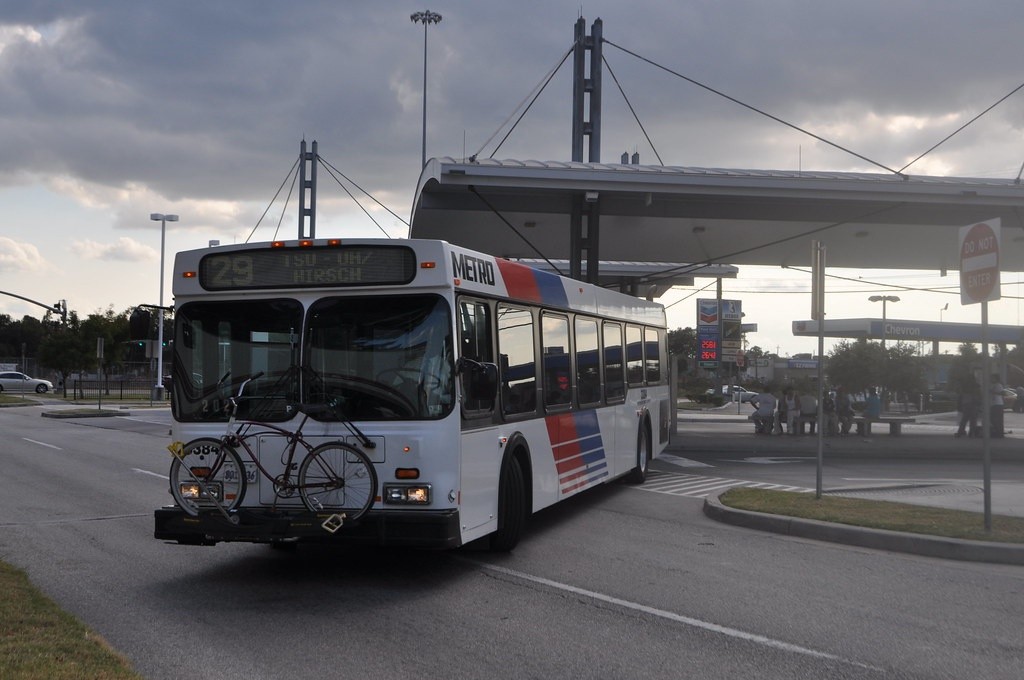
[
  {"xmin": 989, "ymin": 375, "xmax": 1006, "ymax": 438},
  {"xmin": 751, "ymin": 386, "xmax": 777, "ymax": 432},
  {"xmin": 772, "ymin": 386, "xmax": 801, "ymax": 435},
  {"xmin": 957, "ymin": 373, "xmax": 979, "ymax": 437},
  {"xmin": 391, "ymin": 337, "xmax": 451, "ymax": 413},
  {"xmin": 799, "ymin": 393, "xmax": 817, "ymax": 434},
  {"xmin": 858, "ymin": 387, "xmax": 879, "ymax": 421},
  {"xmin": 1016, "ymin": 383, "xmax": 1024, "ymax": 412},
  {"xmin": 822, "ymin": 390, "xmax": 838, "ymax": 435},
  {"xmin": 835, "ymin": 387, "xmax": 853, "ymax": 437}
]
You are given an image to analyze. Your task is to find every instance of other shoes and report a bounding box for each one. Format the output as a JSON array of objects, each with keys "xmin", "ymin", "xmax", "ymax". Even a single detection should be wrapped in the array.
[
  {"xmin": 771, "ymin": 430, "xmax": 781, "ymax": 435},
  {"xmin": 787, "ymin": 430, "xmax": 793, "ymax": 434}
]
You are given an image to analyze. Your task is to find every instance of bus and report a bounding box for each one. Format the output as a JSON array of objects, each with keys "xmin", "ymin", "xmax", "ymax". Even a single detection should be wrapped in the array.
[{"xmin": 127, "ymin": 239, "xmax": 676, "ymax": 554}]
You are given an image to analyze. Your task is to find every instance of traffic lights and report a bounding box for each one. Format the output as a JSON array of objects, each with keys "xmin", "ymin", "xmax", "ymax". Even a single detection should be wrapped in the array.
[
  {"xmin": 162, "ymin": 340, "xmax": 167, "ymax": 347},
  {"xmin": 137, "ymin": 341, "xmax": 144, "ymax": 352}
]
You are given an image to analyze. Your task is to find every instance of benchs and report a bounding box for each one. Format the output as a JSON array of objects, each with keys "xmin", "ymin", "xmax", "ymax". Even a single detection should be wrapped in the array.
[{"xmin": 748, "ymin": 415, "xmax": 916, "ymax": 437}]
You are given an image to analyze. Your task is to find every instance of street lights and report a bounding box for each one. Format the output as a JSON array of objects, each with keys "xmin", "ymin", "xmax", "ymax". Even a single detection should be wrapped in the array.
[
  {"xmin": 868, "ymin": 295, "xmax": 896, "ymax": 346},
  {"xmin": 408, "ymin": 8, "xmax": 442, "ymax": 169},
  {"xmin": 151, "ymin": 214, "xmax": 178, "ymax": 400}
]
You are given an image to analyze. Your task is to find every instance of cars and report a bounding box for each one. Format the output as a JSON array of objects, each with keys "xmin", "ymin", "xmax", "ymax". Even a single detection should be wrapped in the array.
[
  {"xmin": 1003, "ymin": 387, "xmax": 1023, "ymax": 412},
  {"xmin": 706, "ymin": 384, "xmax": 759, "ymax": 403},
  {"xmin": 0, "ymin": 370, "xmax": 54, "ymax": 394}
]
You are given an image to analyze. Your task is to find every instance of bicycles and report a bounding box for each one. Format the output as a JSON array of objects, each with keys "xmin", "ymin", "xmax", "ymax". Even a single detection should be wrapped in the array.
[{"xmin": 168, "ymin": 371, "xmax": 379, "ymax": 532}]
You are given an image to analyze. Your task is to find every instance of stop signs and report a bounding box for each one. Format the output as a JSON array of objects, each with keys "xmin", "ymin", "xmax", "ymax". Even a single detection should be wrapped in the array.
[{"xmin": 958, "ymin": 223, "xmax": 999, "ymax": 300}]
